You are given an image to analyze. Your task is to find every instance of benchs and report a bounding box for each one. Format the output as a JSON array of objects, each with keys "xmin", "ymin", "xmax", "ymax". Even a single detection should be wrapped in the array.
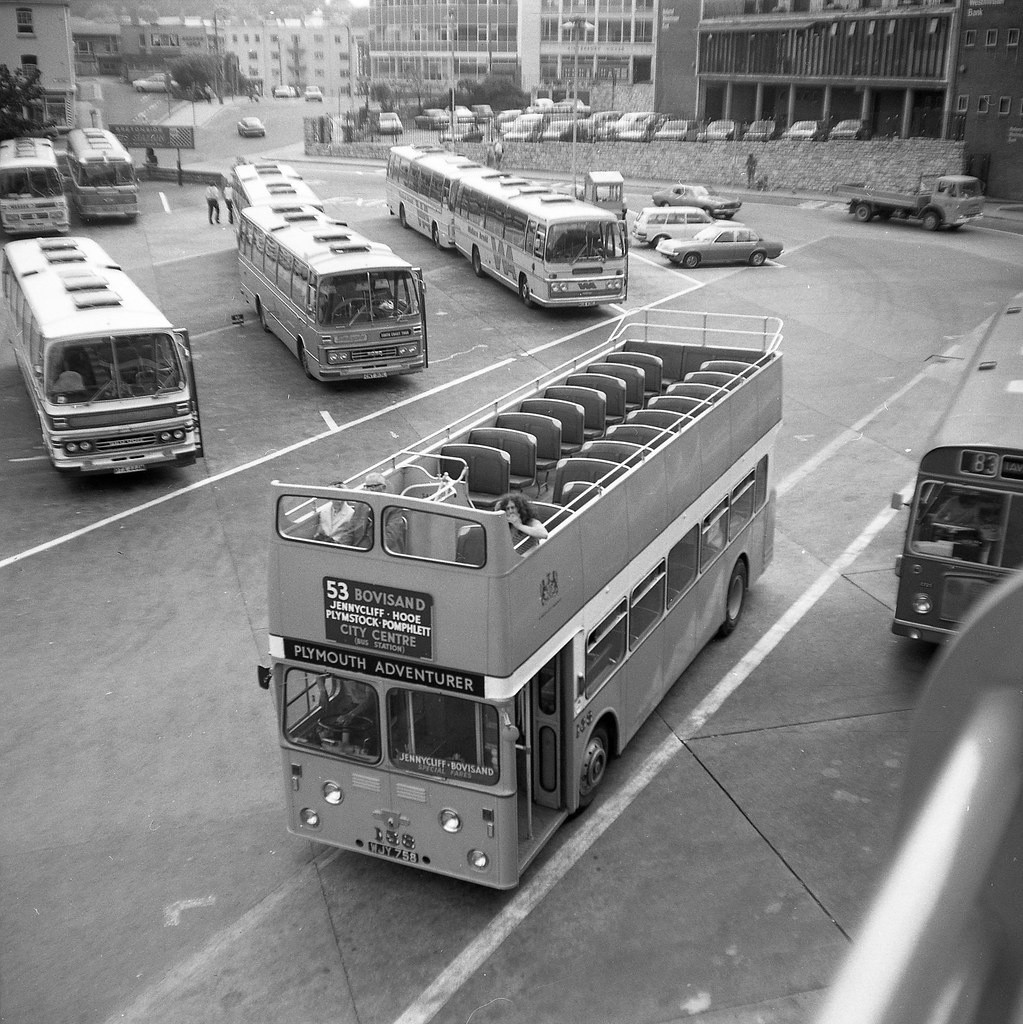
[{"xmin": 315, "ymin": 339, "xmax": 779, "ymax": 760}]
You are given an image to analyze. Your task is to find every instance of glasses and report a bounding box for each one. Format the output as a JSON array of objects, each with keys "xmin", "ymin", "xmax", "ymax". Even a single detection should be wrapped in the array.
[{"xmin": 363, "ymin": 482, "xmax": 382, "ymax": 488}]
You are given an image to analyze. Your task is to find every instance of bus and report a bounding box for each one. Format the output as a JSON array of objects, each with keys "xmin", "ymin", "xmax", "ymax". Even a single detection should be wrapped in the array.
[
  {"xmin": 230, "ymin": 158, "xmax": 325, "ymax": 243},
  {"xmin": 236, "ymin": 202, "xmax": 429, "ymax": 382},
  {"xmin": 383, "ymin": 142, "xmax": 631, "ymax": 310},
  {"xmin": 254, "ymin": 306, "xmax": 783, "ymax": 890},
  {"xmin": 1, "ymin": 137, "xmax": 72, "ymax": 238},
  {"xmin": 1, "ymin": 235, "xmax": 203, "ymax": 477},
  {"xmin": 887, "ymin": 297, "xmax": 1023, "ymax": 655},
  {"xmin": 64, "ymin": 127, "xmax": 140, "ymax": 222}
]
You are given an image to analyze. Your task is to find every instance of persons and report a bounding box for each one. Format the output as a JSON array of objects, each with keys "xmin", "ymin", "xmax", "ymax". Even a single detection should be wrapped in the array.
[
  {"xmin": 314, "ymin": 673, "xmax": 400, "ymax": 743},
  {"xmin": 206, "ymin": 179, "xmax": 221, "ymax": 224},
  {"xmin": 491, "ymin": 492, "xmax": 548, "ymax": 557},
  {"xmin": 314, "ymin": 472, "xmax": 406, "ymax": 551},
  {"xmin": 745, "ymin": 149, "xmax": 760, "ymax": 191},
  {"xmin": 932, "ymin": 490, "xmax": 986, "ymax": 535},
  {"xmin": 485, "ymin": 137, "xmax": 505, "ymax": 170},
  {"xmin": 203, "ymin": 82, "xmax": 214, "ymax": 106},
  {"xmin": 223, "ymin": 183, "xmax": 234, "ymax": 224}
]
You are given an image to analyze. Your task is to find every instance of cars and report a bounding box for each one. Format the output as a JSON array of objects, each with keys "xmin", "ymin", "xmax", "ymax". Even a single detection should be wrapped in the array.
[
  {"xmin": 131, "ymin": 73, "xmax": 182, "ymax": 94},
  {"xmin": 631, "ymin": 205, "xmax": 785, "ymax": 269},
  {"xmin": 652, "ymin": 183, "xmax": 742, "ymax": 219},
  {"xmin": 275, "ymin": 84, "xmax": 322, "ymax": 102},
  {"xmin": 414, "ymin": 98, "xmax": 661, "ymax": 142},
  {"xmin": 655, "ymin": 118, "xmax": 873, "ymax": 141},
  {"xmin": 236, "ymin": 117, "xmax": 265, "ymax": 137},
  {"xmin": 375, "ymin": 112, "xmax": 403, "ymax": 135}
]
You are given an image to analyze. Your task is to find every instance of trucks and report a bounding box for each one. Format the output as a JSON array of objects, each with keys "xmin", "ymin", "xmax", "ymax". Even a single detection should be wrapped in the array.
[{"xmin": 833, "ymin": 171, "xmax": 986, "ymax": 232}]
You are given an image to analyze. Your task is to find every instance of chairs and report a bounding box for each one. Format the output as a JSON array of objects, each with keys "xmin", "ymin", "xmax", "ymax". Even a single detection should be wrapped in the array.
[{"xmin": 95, "ymin": 336, "xmax": 163, "ymax": 380}]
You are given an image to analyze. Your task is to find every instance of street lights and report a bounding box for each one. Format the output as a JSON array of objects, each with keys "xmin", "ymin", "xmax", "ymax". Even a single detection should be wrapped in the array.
[{"xmin": 562, "ymin": 20, "xmax": 596, "ymax": 179}]
